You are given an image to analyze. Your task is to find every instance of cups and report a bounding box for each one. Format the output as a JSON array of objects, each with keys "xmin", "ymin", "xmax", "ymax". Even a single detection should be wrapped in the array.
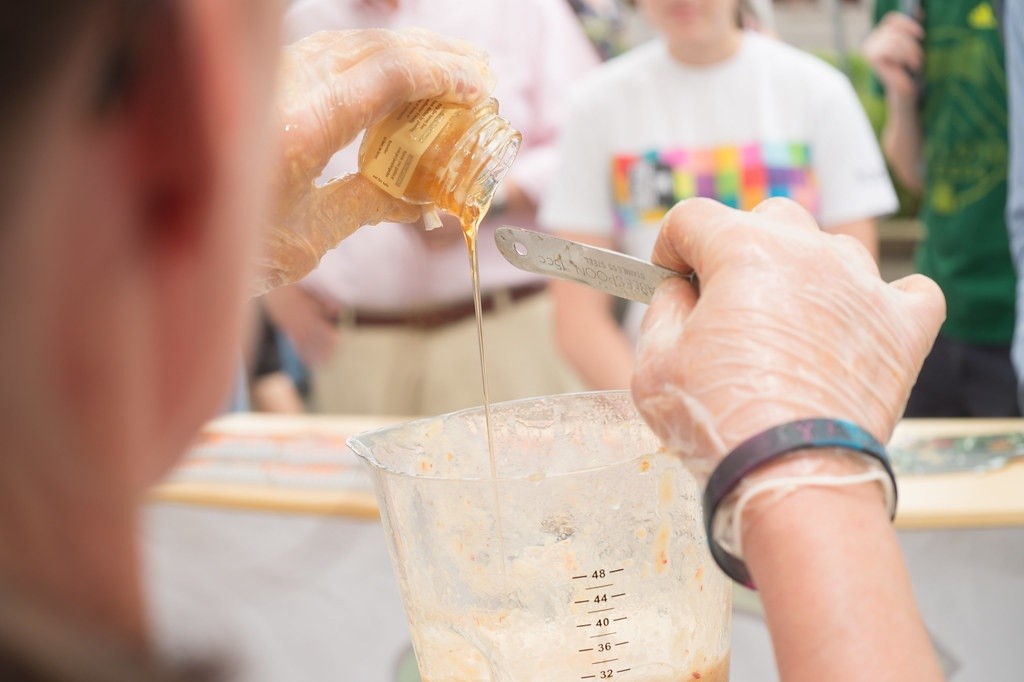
[{"xmin": 345, "ymin": 387, "xmax": 732, "ymax": 682}]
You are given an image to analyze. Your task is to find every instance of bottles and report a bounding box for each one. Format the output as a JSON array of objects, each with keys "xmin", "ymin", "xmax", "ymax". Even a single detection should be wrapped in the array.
[{"xmin": 358, "ymin": 90, "xmax": 523, "ymax": 223}]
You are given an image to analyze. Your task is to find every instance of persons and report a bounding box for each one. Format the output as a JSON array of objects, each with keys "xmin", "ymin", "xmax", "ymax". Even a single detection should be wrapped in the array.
[
  {"xmin": 0, "ymin": 0, "xmax": 965, "ymax": 682},
  {"xmin": 258, "ymin": 0, "xmax": 604, "ymax": 425},
  {"xmin": 534, "ymin": 0, "xmax": 900, "ymax": 391},
  {"xmin": 863, "ymin": 0, "xmax": 1024, "ymax": 418}
]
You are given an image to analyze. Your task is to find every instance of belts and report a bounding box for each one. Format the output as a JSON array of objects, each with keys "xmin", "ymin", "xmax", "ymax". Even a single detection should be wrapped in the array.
[{"xmin": 330, "ymin": 281, "xmax": 551, "ymax": 331}]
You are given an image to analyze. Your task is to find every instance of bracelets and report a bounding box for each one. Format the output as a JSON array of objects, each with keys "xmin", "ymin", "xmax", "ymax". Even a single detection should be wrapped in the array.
[{"xmin": 703, "ymin": 416, "xmax": 899, "ymax": 589}]
